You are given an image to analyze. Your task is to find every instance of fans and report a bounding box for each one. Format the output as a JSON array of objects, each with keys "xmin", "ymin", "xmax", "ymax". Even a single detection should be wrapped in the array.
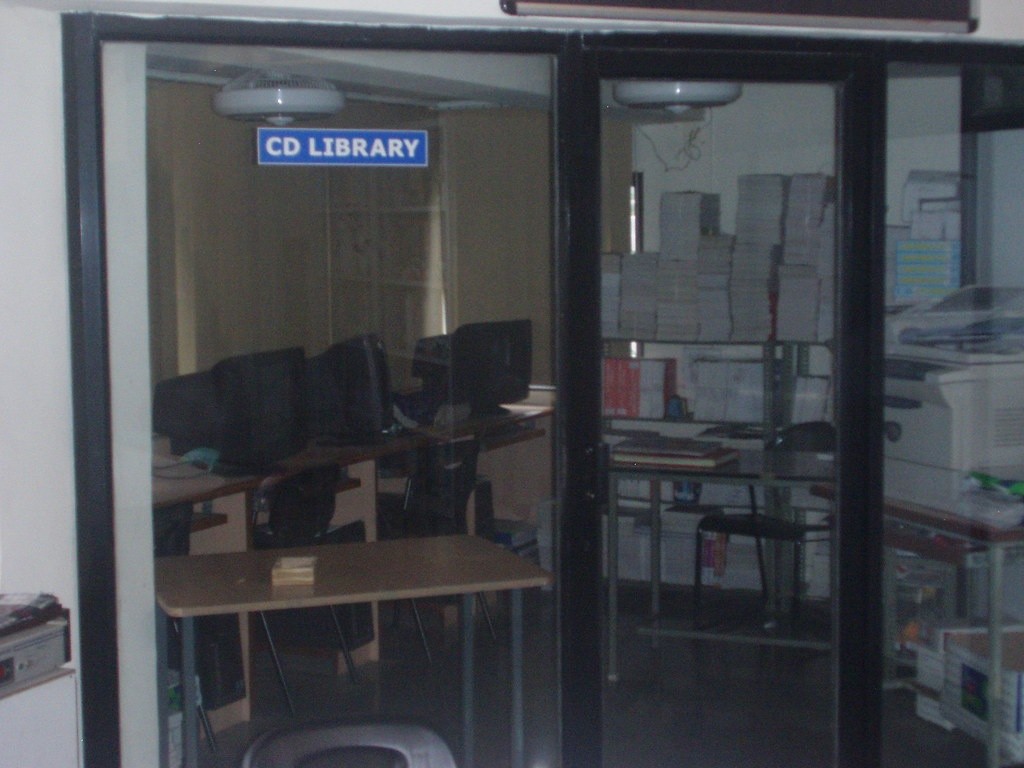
[
  {"xmin": 212, "ymin": 70, "xmax": 342, "ymax": 126},
  {"xmin": 613, "ymin": 78, "xmax": 742, "ymax": 117}
]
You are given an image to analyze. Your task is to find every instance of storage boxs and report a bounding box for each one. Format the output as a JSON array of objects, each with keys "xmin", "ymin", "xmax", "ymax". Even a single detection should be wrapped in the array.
[
  {"xmin": 695, "ymin": 359, "xmax": 764, "ymax": 423},
  {"xmin": 883, "ymin": 169, "xmax": 965, "ymax": 298},
  {"xmin": 167, "ymin": 672, "xmax": 203, "ymax": 767},
  {"xmin": 604, "ymin": 356, "xmax": 678, "ymax": 417},
  {"xmin": 890, "ymin": 554, "xmax": 1024, "ymax": 764},
  {"xmin": 606, "ymin": 173, "xmax": 839, "ymax": 345}
]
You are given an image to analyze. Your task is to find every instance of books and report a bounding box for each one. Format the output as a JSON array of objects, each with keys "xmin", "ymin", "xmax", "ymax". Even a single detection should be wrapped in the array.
[
  {"xmin": 791, "ymin": 374, "xmax": 834, "ymax": 424},
  {"xmin": 602, "ymin": 505, "xmax": 831, "ymax": 598},
  {"xmin": 601, "ymin": 173, "xmax": 833, "ymax": 340},
  {"xmin": 612, "ymin": 437, "xmax": 740, "ymax": 467},
  {"xmin": 600, "ymin": 355, "xmax": 677, "ymax": 419}
]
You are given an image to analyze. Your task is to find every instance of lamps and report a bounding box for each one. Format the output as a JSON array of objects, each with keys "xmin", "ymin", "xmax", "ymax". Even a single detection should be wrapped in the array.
[{"xmin": 501, "ymin": 1, "xmax": 981, "ymax": 37}]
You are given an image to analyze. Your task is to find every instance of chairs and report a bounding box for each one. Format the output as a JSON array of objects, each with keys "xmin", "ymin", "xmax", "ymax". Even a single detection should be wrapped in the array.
[
  {"xmin": 375, "ymin": 438, "xmax": 499, "ymax": 666},
  {"xmin": 153, "ymin": 503, "xmax": 217, "ymax": 752},
  {"xmin": 249, "ymin": 464, "xmax": 363, "ymax": 711},
  {"xmin": 690, "ymin": 423, "xmax": 839, "ymax": 631}
]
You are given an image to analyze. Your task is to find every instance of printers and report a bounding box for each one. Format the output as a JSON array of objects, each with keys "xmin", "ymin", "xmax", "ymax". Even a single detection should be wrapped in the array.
[{"xmin": 885, "ymin": 284, "xmax": 1024, "ymax": 514}]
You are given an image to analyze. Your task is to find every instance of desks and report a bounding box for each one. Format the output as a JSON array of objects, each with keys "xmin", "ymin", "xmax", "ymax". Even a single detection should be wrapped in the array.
[
  {"xmin": 602, "ymin": 444, "xmax": 842, "ymax": 682},
  {"xmin": 153, "ymin": 404, "xmax": 558, "ymax": 744},
  {"xmin": 885, "ymin": 500, "xmax": 1024, "ymax": 767},
  {"xmin": 155, "ymin": 534, "xmax": 551, "ymax": 768}
]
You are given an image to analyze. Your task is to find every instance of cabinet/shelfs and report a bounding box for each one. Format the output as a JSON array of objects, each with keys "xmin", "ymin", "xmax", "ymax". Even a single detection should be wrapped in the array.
[{"xmin": 326, "ymin": 165, "xmax": 453, "ymax": 391}]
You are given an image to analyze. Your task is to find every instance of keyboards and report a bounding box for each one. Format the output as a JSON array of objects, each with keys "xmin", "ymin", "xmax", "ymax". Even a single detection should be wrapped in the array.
[{"xmin": 610, "ymin": 434, "xmax": 724, "ymax": 457}]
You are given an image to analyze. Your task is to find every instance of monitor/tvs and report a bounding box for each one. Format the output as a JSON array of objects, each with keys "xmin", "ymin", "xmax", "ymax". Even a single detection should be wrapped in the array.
[
  {"xmin": 412, "ymin": 318, "xmax": 532, "ymax": 417},
  {"xmin": 151, "ymin": 332, "xmax": 395, "ymax": 475}
]
